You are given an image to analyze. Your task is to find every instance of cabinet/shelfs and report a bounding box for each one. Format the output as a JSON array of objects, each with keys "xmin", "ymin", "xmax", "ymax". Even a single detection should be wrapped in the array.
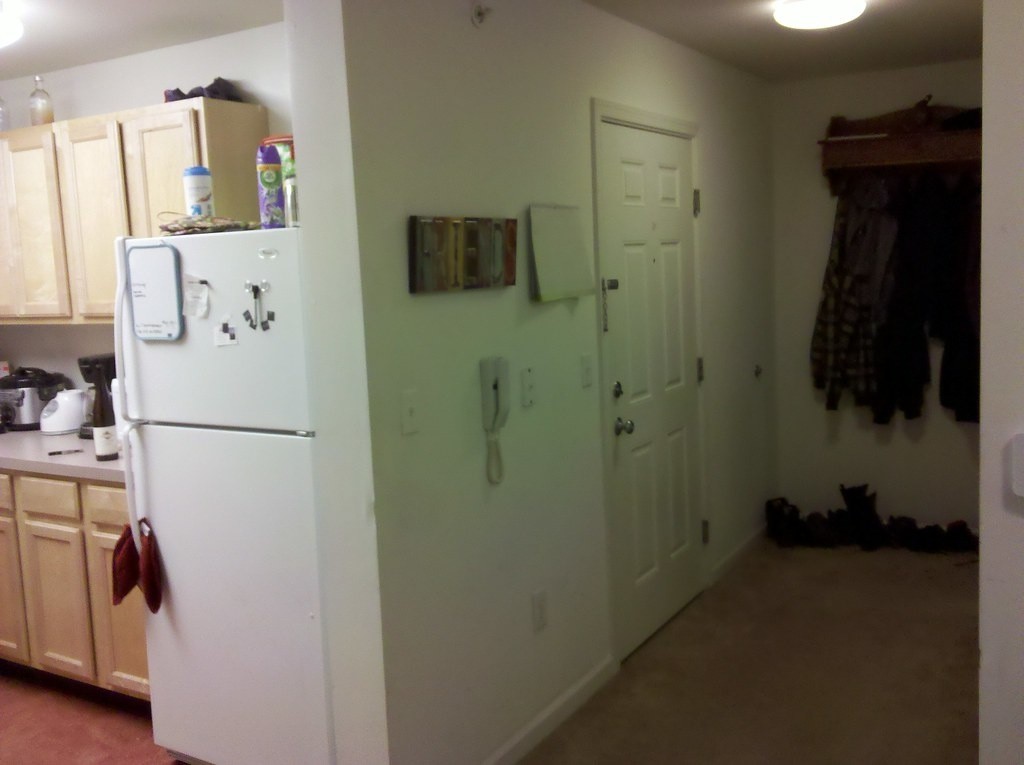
[{"xmin": 1, "ymin": 96, "xmax": 276, "ymax": 711}]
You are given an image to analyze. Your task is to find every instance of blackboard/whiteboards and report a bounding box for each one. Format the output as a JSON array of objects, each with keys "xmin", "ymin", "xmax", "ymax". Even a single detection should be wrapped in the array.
[{"xmin": 125, "ymin": 244, "xmax": 184, "ymax": 342}]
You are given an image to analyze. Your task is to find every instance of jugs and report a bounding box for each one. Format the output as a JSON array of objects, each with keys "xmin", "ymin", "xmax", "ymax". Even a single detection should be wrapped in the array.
[{"xmin": 79, "ymin": 386, "xmax": 96, "ymax": 438}]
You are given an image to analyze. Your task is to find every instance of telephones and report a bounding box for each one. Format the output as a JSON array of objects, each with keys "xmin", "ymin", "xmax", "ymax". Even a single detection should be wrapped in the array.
[{"xmin": 479, "ymin": 354, "xmax": 510, "ymax": 432}]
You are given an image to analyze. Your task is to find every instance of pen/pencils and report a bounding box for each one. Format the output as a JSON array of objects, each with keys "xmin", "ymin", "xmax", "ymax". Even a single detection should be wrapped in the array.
[{"xmin": 48, "ymin": 449, "xmax": 83, "ymax": 456}]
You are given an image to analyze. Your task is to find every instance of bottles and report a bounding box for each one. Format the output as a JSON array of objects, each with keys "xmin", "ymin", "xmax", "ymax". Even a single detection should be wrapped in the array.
[
  {"xmin": 181, "ymin": 165, "xmax": 215, "ymax": 220},
  {"xmin": 282, "ymin": 173, "xmax": 299, "ymax": 227},
  {"xmin": 252, "ymin": 145, "xmax": 284, "ymax": 229},
  {"xmin": 89, "ymin": 365, "xmax": 119, "ymax": 460},
  {"xmin": 25, "ymin": 74, "xmax": 54, "ymax": 126},
  {"xmin": 0, "ymin": 97, "xmax": 10, "ymax": 130}
]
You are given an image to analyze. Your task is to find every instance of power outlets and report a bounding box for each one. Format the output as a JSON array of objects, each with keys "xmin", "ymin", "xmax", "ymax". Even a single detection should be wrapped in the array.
[
  {"xmin": 520, "ymin": 365, "xmax": 539, "ymax": 409},
  {"xmin": 529, "ymin": 591, "xmax": 552, "ymax": 635},
  {"xmin": 399, "ymin": 386, "xmax": 427, "ymax": 437}
]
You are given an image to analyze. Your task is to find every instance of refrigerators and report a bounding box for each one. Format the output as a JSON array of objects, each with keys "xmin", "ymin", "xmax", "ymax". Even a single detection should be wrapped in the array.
[{"xmin": 112, "ymin": 229, "xmax": 336, "ymax": 765}]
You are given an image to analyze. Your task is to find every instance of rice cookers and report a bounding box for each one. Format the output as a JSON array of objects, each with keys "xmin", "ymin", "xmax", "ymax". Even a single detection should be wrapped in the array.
[{"xmin": 0, "ymin": 366, "xmax": 66, "ymax": 431}]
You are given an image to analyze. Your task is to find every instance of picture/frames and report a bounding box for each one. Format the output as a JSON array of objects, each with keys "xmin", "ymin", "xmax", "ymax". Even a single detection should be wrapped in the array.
[{"xmin": 409, "ymin": 212, "xmax": 521, "ymax": 295}]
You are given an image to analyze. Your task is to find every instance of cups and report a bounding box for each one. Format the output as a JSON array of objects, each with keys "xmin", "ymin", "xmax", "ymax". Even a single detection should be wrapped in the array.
[{"xmin": 0, "ymin": 402, "xmax": 16, "ymax": 433}]
